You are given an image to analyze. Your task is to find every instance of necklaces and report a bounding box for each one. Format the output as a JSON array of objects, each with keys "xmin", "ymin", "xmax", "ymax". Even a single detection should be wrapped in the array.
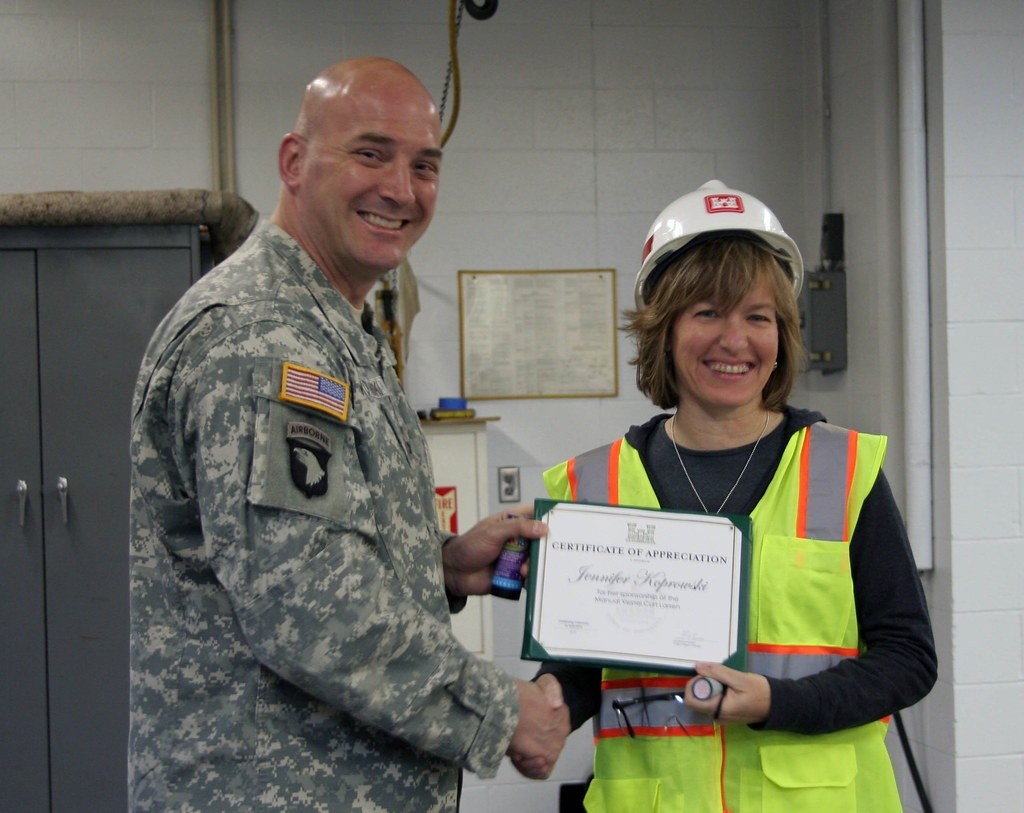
[{"xmin": 671, "ymin": 410, "xmax": 769, "ymax": 514}]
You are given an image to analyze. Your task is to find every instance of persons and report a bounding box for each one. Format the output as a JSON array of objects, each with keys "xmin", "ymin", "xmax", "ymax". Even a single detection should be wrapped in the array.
[
  {"xmin": 511, "ymin": 180, "xmax": 939, "ymax": 813},
  {"xmin": 127, "ymin": 56, "xmax": 570, "ymax": 813}
]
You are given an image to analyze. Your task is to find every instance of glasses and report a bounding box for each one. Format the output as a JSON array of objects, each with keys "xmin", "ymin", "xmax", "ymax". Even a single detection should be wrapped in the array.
[{"xmin": 612, "ymin": 691, "xmax": 713, "ymax": 739}]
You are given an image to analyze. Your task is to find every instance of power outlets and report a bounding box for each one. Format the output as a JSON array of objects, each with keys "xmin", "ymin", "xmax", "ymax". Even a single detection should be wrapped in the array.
[{"xmin": 498, "ymin": 466, "xmax": 521, "ymax": 503}]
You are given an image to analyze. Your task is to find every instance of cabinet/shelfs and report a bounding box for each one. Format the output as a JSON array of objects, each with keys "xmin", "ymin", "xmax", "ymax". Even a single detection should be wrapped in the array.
[{"xmin": 0, "ymin": 221, "xmax": 225, "ymax": 813}]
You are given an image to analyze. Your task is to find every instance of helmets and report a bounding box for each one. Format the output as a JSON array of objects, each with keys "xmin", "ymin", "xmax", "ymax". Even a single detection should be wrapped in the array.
[{"xmin": 634, "ymin": 179, "xmax": 804, "ymax": 311}]
